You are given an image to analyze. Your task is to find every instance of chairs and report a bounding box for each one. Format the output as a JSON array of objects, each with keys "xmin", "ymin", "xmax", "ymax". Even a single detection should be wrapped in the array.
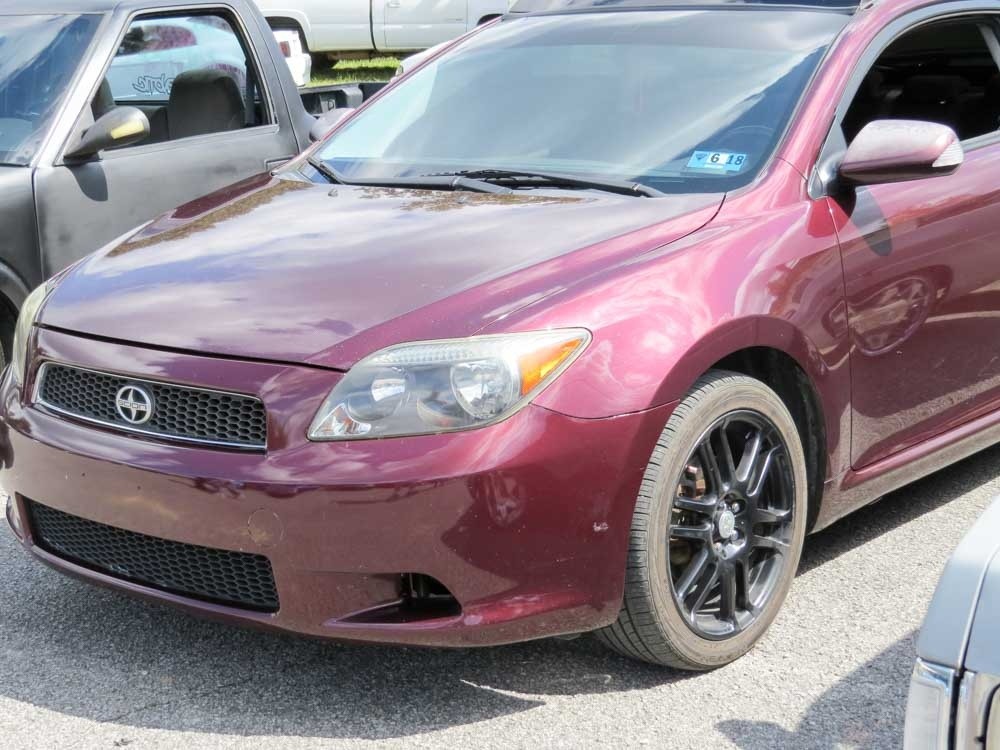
[
  {"xmin": 168, "ymin": 69, "xmax": 245, "ymax": 140},
  {"xmin": 90, "ymin": 77, "xmax": 114, "ymax": 121}
]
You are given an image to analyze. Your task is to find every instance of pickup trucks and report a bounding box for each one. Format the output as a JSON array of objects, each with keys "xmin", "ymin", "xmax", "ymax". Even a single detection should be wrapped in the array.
[{"xmin": 0, "ymin": 1, "xmax": 378, "ymax": 380}]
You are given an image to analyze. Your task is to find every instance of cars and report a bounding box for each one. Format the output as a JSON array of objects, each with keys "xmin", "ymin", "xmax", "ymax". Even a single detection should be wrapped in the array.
[
  {"xmin": 0, "ymin": 1, "xmax": 1000, "ymax": 674},
  {"xmin": 903, "ymin": 494, "xmax": 999, "ymax": 750},
  {"xmin": 96, "ymin": 16, "xmax": 312, "ymax": 109},
  {"xmin": 243, "ymin": 0, "xmax": 516, "ymax": 86}
]
